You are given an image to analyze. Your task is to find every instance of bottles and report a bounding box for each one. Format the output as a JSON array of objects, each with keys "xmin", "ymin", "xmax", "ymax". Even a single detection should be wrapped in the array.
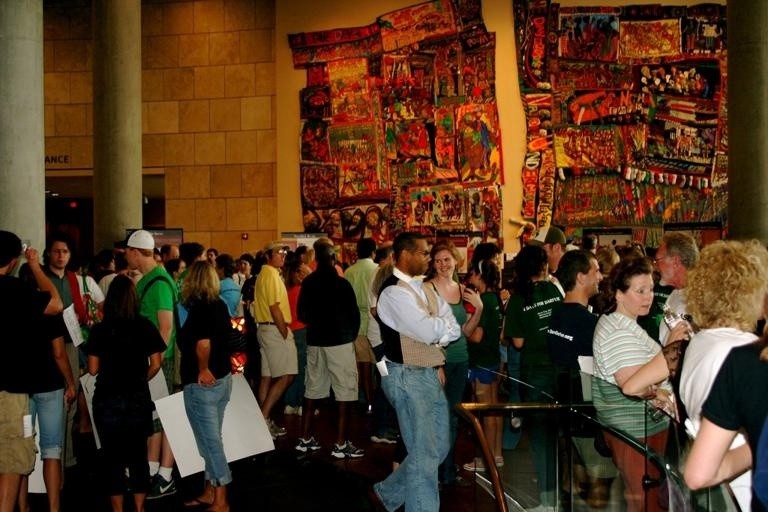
[
  {"xmin": 661, "ymin": 303, "xmax": 695, "ymax": 341},
  {"xmin": 463, "ymin": 283, "xmax": 476, "ymax": 313}
]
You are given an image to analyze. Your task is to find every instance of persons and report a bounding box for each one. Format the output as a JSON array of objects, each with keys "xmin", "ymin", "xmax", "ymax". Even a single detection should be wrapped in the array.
[
  {"xmin": 678, "ymin": 240, "xmax": 768, "ymax": 512},
  {"xmin": 175, "ymin": 261, "xmax": 235, "ymax": 511},
  {"xmin": 97, "ymin": 259, "xmax": 138, "ymax": 300},
  {"xmin": 343, "ymin": 236, "xmax": 381, "ymax": 414},
  {"xmin": 295, "ymin": 245, "xmax": 315, "ymax": 264},
  {"xmin": 464, "ymin": 259, "xmax": 505, "ymax": 472},
  {"xmin": 241, "ymin": 257, "xmax": 266, "ymax": 380},
  {"xmin": 656, "ymin": 231, "xmax": 697, "ymax": 345},
  {"xmin": 126, "ymin": 230, "xmax": 180, "ymax": 499},
  {"xmin": 92, "ymin": 253, "xmax": 115, "ymax": 281},
  {"xmin": 637, "ymin": 279, "xmax": 675, "ymax": 341},
  {"xmin": 167, "ymin": 259, "xmax": 186, "ymax": 387},
  {"xmin": 232, "ymin": 254, "xmax": 253, "ymax": 316},
  {"xmin": 683, "ymin": 288, "xmax": 767, "ymax": 511},
  {"xmin": 206, "ymin": 248, "xmax": 218, "ymax": 267},
  {"xmin": 506, "ymin": 246, "xmax": 562, "ymax": 511},
  {"xmin": 365, "ymin": 231, "xmax": 462, "ymax": 512},
  {"xmin": 299, "ymin": 236, "xmax": 344, "ymax": 281},
  {"xmin": 545, "ymin": 250, "xmax": 618, "ymax": 511},
  {"xmin": 472, "ymin": 243, "xmax": 506, "ymax": 384},
  {"xmin": 566, "ymin": 234, "xmax": 598, "ymax": 254},
  {"xmin": 18, "ymin": 261, "xmax": 77, "ymax": 512},
  {"xmin": 67, "ymin": 266, "xmax": 105, "ymax": 436},
  {"xmin": 0, "ymin": 227, "xmax": 65, "ymax": 512},
  {"xmin": 422, "ymin": 239, "xmax": 484, "ymax": 486},
  {"xmin": 180, "ymin": 241, "xmax": 204, "ymax": 284},
  {"xmin": 160, "ymin": 244, "xmax": 179, "ymax": 260},
  {"xmin": 366, "ymin": 247, "xmax": 392, "ymax": 363},
  {"xmin": 282, "ymin": 251, "xmax": 320, "ymax": 417},
  {"xmin": 619, "ymin": 241, "xmax": 646, "ymax": 257},
  {"xmin": 215, "ymin": 254, "xmax": 241, "ymax": 317},
  {"xmin": 624, "ymin": 338, "xmax": 690, "ymax": 431},
  {"xmin": 296, "ymin": 243, "xmax": 366, "ymax": 461},
  {"xmin": 40, "ymin": 239, "xmax": 85, "ymax": 473},
  {"xmin": 595, "ymin": 247, "xmax": 620, "ymax": 276},
  {"xmin": 524, "ymin": 225, "xmax": 566, "ymax": 299},
  {"xmin": 87, "ymin": 275, "xmax": 167, "ymax": 512},
  {"xmin": 251, "ymin": 241, "xmax": 299, "ymax": 439},
  {"xmin": 592, "ymin": 255, "xmax": 692, "ymax": 511}
]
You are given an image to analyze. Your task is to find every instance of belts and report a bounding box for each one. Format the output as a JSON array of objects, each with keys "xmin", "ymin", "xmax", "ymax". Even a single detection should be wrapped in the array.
[{"xmin": 258, "ymin": 321, "xmax": 276, "ymax": 325}]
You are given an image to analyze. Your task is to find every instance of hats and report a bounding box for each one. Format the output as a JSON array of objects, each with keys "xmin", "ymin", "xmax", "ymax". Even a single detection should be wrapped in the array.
[
  {"xmin": 263, "ymin": 240, "xmax": 290, "ymax": 253},
  {"xmin": 126, "ymin": 229, "xmax": 156, "ymax": 250},
  {"xmin": 524, "ymin": 225, "xmax": 567, "ymax": 247}
]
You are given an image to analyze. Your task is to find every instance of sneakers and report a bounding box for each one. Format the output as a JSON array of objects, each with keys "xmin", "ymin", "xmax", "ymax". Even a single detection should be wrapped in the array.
[
  {"xmin": 265, "ymin": 419, "xmax": 277, "ymax": 440},
  {"xmin": 145, "ymin": 474, "xmax": 177, "ymax": 500},
  {"xmin": 294, "ymin": 435, "xmax": 321, "ymax": 453},
  {"xmin": 462, "ymin": 457, "xmax": 487, "ymax": 472},
  {"xmin": 370, "ymin": 430, "xmax": 398, "ymax": 444},
  {"xmin": 330, "ymin": 439, "xmax": 366, "ymax": 459},
  {"xmin": 493, "ymin": 456, "xmax": 505, "ymax": 467},
  {"xmin": 268, "ymin": 418, "xmax": 287, "ymax": 436}
]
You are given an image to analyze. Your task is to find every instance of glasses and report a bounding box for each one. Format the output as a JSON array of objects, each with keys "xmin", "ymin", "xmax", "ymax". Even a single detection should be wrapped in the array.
[
  {"xmin": 653, "ymin": 256, "xmax": 664, "ymax": 263},
  {"xmin": 406, "ymin": 249, "xmax": 430, "ymax": 256},
  {"xmin": 277, "ymin": 249, "xmax": 288, "ymax": 254}
]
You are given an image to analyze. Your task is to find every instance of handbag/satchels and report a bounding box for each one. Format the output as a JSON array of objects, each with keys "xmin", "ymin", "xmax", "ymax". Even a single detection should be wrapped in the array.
[{"xmin": 82, "ymin": 276, "xmax": 101, "ymax": 326}]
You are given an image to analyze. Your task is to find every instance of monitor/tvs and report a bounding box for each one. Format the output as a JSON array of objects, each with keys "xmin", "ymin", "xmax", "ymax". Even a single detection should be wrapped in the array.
[
  {"xmin": 125, "ymin": 227, "xmax": 185, "ymax": 252},
  {"xmin": 280, "ymin": 231, "xmax": 329, "ymax": 250},
  {"xmin": 663, "ymin": 221, "xmax": 723, "ymax": 251},
  {"xmin": 582, "ymin": 228, "xmax": 633, "ymax": 250}
]
